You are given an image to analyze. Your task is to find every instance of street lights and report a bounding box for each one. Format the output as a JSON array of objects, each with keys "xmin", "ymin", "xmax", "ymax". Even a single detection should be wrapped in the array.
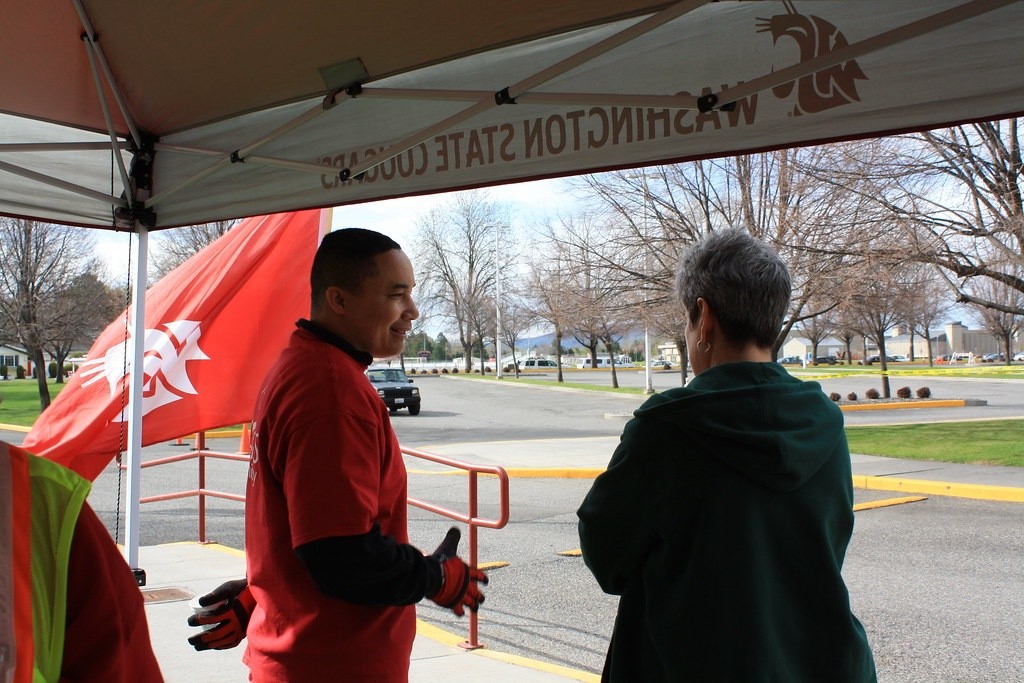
[{"xmin": 484, "ymin": 220, "xmax": 511, "ymax": 383}]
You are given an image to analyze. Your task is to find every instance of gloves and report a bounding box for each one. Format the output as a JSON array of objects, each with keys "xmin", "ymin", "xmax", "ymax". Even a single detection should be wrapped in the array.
[
  {"xmin": 187, "ymin": 579, "xmax": 257, "ymax": 651},
  {"xmin": 425, "ymin": 527, "xmax": 488, "ymax": 617}
]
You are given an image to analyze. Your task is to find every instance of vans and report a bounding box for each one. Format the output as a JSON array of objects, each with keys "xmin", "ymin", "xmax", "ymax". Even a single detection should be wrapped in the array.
[
  {"xmin": 519, "ymin": 359, "xmax": 558, "ymax": 369},
  {"xmin": 583, "ymin": 358, "xmax": 624, "ymax": 368}
]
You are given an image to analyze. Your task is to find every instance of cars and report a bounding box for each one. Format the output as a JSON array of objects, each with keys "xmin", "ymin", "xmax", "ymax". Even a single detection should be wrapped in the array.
[
  {"xmin": 643, "ymin": 360, "xmax": 672, "ymax": 368},
  {"xmin": 364, "ymin": 368, "xmax": 421, "ymax": 415},
  {"xmin": 891, "ymin": 353, "xmax": 911, "ymax": 362},
  {"xmin": 776, "ymin": 357, "xmax": 803, "ymax": 364},
  {"xmin": 809, "ymin": 357, "xmax": 839, "ymax": 364},
  {"xmin": 861, "ymin": 355, "xmax": 895, "ymax": 362},
  {"xmin": 935, "ymin": 351, "xmax": 1024, "ymax": 362}
]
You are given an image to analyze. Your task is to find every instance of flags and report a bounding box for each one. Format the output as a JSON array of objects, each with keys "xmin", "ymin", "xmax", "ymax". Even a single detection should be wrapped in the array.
[{"xmin": 16, "ymin": 206, "xmax": 335, "ymax": 484}]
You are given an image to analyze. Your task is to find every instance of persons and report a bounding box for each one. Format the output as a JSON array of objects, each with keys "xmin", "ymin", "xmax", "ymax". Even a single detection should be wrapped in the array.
[
  {"xmin": 189, "ymin": 229, "xmax": 491, "ymax": 683},
  {"xmin": 0, "ymin": 441, "xmax": 166, "ymax": 683},
  {"xmin": 578, "ymin": 227, "xmax": 877, "ymax": 683}
]
